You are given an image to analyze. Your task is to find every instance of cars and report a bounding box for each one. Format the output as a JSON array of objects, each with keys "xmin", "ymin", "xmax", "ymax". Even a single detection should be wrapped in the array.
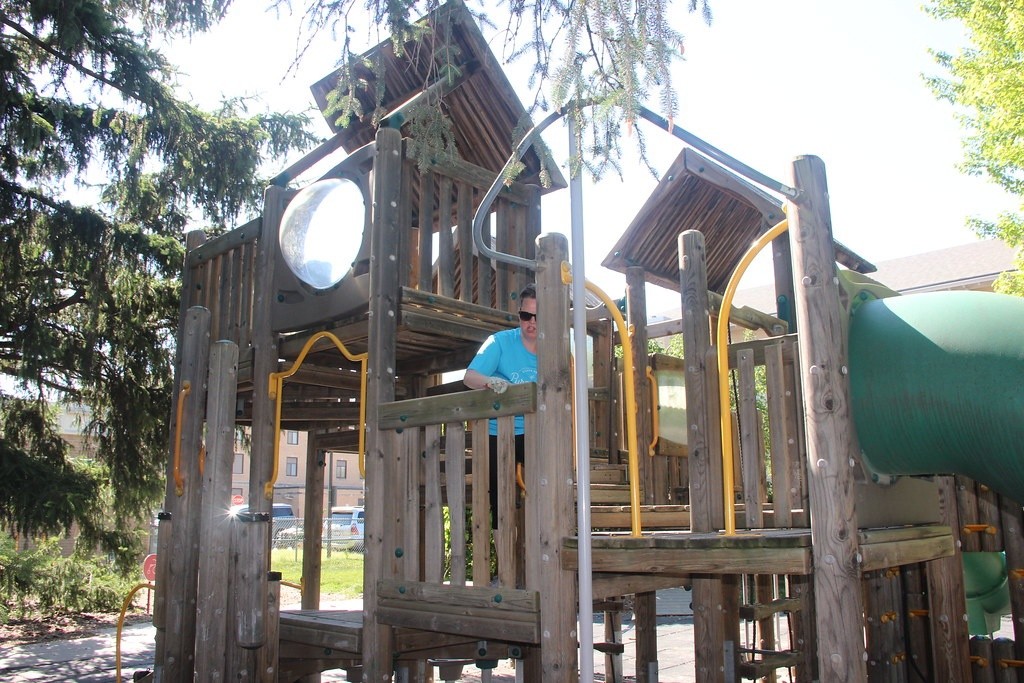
[{"xmin": 276, "ymin": 517, "xmax": 324, "ymax": 550}]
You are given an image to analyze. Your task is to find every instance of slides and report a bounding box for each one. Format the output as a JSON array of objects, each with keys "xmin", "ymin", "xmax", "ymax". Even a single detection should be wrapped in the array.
[{"xmin": 843, "ymin": 288, "xmax": 1024, "ymax": 639}]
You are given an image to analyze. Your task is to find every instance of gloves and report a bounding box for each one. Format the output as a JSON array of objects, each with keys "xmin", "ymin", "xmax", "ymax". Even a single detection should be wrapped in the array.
[{"xmin": 483, "ymin": 376, "xmax": 513, "ymax": 394}]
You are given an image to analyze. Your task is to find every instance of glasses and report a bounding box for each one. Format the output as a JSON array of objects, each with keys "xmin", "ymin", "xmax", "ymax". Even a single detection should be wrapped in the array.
[{"xmin": 518, "ymin": 308, "xmax": 536, "ymax": 322}]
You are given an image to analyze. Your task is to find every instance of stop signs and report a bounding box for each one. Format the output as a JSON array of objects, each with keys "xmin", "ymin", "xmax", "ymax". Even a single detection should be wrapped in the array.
[{"xmin": 232, "ymin": 495, "xmax": 245, "ymax": 507}]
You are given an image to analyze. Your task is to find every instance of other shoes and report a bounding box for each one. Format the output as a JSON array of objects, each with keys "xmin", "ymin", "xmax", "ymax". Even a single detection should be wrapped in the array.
[{"xmin": 491, "ymin": 577, "xmax": 498, "ymax": 587}]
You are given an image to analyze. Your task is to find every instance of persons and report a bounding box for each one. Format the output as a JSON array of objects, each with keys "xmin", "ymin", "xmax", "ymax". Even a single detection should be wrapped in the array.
[{"xmin": 463, "ymin": 283, "xmax": 536, "ymax": 582}]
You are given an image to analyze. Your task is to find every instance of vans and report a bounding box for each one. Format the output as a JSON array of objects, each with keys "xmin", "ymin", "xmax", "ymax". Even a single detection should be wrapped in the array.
[
  {"xmin": 321, "ymin": 505, "xmax": 364, "ymax": 553},
  {"xmin": 234, "ymin": 503, "xmax": 296, "ymax": 546}
]
